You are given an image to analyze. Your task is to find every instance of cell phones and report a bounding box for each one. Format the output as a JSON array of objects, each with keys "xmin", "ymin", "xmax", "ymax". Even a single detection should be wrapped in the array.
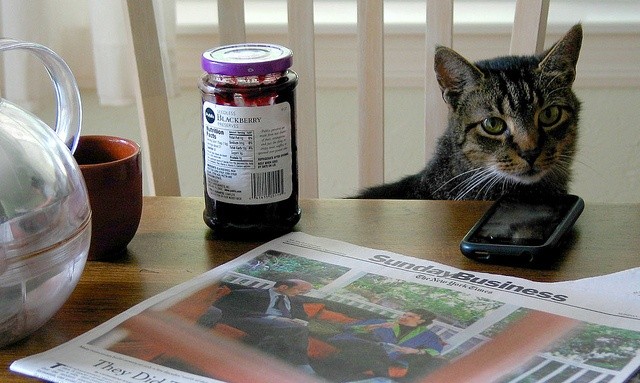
[{"xmin": 459, "ymin": 192, "xmax": 584, "ymax": 265}]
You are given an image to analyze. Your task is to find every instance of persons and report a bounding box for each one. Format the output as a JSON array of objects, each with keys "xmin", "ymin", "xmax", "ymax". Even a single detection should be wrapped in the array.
[
  {"xmin": 312, "ymin": 309, "xmax": 443, "ymax": 383},
  {"xmin": 215, "ymin": 279, "xmax": 315, "ymax": 378}
]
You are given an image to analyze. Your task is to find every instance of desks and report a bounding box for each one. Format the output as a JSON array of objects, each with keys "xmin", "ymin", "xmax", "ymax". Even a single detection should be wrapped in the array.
[{"xmin": 0, "ymin": 197, "xmax": 640, "ymax": 383}]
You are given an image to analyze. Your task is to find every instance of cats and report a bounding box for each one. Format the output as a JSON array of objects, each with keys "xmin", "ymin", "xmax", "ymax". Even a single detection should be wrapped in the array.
[{"xmin": 336, "ymin": 18, "xmax": 585, "ymax": 201}]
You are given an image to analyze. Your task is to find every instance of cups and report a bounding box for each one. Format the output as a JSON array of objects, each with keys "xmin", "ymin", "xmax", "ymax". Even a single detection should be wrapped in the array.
[{"xmin": 73, "ymin": 136, "xmax": 143, "ymax": 261}]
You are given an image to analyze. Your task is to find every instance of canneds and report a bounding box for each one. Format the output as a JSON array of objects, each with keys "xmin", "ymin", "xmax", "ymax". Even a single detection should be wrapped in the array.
[{"xmin": 196, "ymin": 42, "xmax": 303, "ymax": 244}]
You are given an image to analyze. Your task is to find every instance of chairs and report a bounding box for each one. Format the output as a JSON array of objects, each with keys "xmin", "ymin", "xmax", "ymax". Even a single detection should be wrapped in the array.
[{"xmin": 126, "ymin": 0, "xmax": 550, "ymax": 199}]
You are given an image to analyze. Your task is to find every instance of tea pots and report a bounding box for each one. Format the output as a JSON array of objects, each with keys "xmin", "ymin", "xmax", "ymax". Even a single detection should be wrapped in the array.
[{"xmin": 0, "ymin": 38, "xmax": 92, "ymax": 348}]
